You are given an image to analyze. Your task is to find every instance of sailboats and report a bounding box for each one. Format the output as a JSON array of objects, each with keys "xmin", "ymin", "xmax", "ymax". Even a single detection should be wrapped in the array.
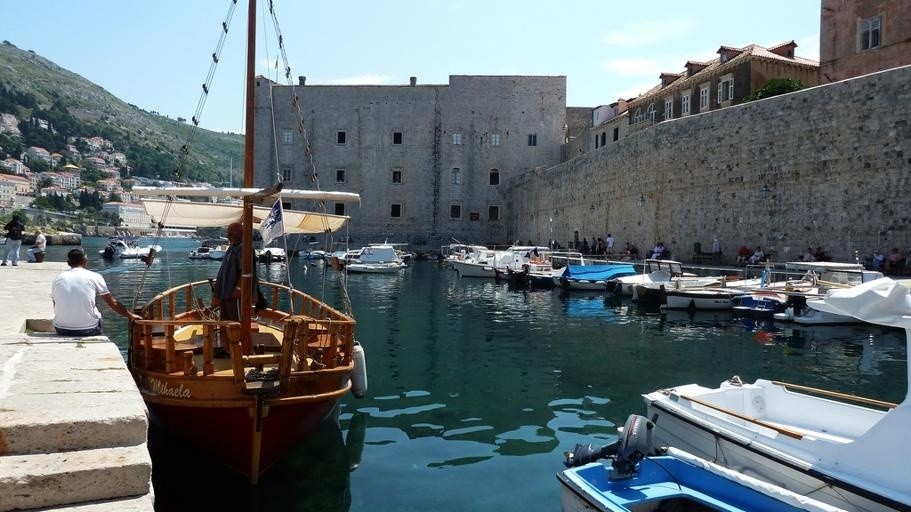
[
  {"xmin": 122, "ymin": 1, "xmax": 377, "ymax": 495},
  {"xmin": 153, "ymin": 412, "xmax": 380, "ymax": 511}
]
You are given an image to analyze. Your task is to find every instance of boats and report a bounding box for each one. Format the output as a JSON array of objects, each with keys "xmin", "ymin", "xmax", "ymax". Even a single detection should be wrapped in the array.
[
  {"xmin": 185, "ymin": 233, "xmax": 415, "ymax": 275},
  {"xmin": 635, "ymin": 273, "xmax": 911, "ymax": 511},
  {"xmin": 552, "ymin": 436, "xmax": 853, "ymax": 512},
  {"xmin": 95, "ymin": 224, "xmax": 163, "ymax": 260}
]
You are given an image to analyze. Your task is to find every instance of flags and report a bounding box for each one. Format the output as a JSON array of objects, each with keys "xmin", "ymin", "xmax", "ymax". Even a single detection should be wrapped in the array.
[{"xmin": 258, "ymin": 197, "xmax": 285, "ymax": 243}]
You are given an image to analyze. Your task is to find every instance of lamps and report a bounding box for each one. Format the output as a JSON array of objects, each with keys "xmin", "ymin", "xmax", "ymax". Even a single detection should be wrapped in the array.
[
  {"xmin": 758, "ymin": 179, "xmax": 776, "ymax": 199},
  {"xmin": 637, "ymin": 191, "xmax": 653, "ymax": 208}
]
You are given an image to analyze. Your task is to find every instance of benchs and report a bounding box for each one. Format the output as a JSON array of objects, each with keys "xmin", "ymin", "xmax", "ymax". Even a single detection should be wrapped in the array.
[
  {"xmin": 797, "ymin": 253, "xmax": 832, "ymax": 262},
  {"xmin": 690, "ymin": 250, "xmax": 722, "ymax": 266},
  {"xmin": 861, "ymin": 255, "xmax": 907, "ymax": 276},
  {"xmin": 643, "ymin": 248, "xmax": 669, "ymax": 259},
  {"xmin": 734, "ymin": 251, "xmax": 773, "ymax": 268}
]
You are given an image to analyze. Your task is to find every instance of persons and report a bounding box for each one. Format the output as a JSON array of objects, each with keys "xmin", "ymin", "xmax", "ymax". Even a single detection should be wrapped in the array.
[
  {"xmin": 871, "ymin": 247, "xmax": 906, "ymax": 273},
  {"xmin": 801, "ymin": 246, "xmax": 828, "ymax": 262},
  {"xmin": 52, "ymin": 249, "xmax": 143, "ymax": 337},
  {"xmin": 213, "ymin": 223, "xmax": 256, "ymax": 359},
  {"xmin": 552, "ymin": 234, "xmax": 615, "ymax": 260},
  {"xmin": 619, "ymin": 241, "xmax": 665, "ymax": 261},
  {"xmin": 27, "ymin": 229, "xmax": 47, "ymax": 263},
  {"xmin": 711, "ymin": 239, "xmax": 765, "ymax": 268},
  {"xmin": 1, "ymin": 213, "xmax": 25, "ymax": 266}
]
